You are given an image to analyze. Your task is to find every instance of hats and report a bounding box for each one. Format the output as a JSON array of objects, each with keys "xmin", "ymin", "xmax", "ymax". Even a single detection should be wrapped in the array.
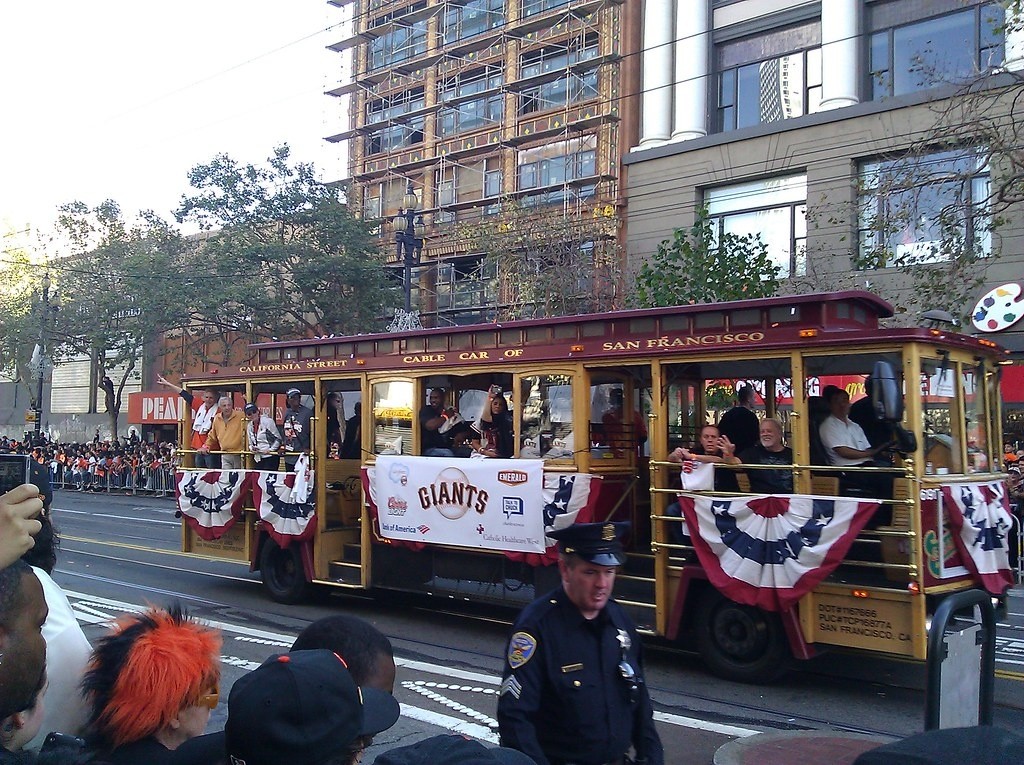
[
  {"xmin": 545, "ymin": 521, "xmax": 632, "ymax": 566},
  {"xmin": 285, "ymin": 387, "xmax": 301, "ymax": 399},
  {"xmin": 244, "ymin": 403, "xmax": 258, "ymax": 413},
  {"xmin": 225, "ymin": 648, "xmax": 401, "ymax": 765}
]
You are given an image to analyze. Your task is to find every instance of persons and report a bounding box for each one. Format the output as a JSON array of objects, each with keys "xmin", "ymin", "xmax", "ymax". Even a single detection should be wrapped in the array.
[
  {"xmin": 20, "ymin": 459, "xmax": 99, "ymax": 750},
  {"xmin": 374, "ymin": 734, "xmax": 535, "ymax": 765},
  {"xmin": 0, "ymin": 484, "xmax": 84, "ymax": 765},
  {"xmin": 0, "ymin": 374, "xmax": 1024, "ymax": 572},
  {"xmin": 224, "ymin": 649, "xmax": 400, "ymax": 765},
  {"xmin": 77, "ymin": 597, "xmax": 224, "ymax": 765},
  {"xmin": 167, "ymin": 616, "xmax": 395, "ymax": 765},
  {"xmin": 497, "ymin": 521, "xmax": 664, "ymax": 765}
]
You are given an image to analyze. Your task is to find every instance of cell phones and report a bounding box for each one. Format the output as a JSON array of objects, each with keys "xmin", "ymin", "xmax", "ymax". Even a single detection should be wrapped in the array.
[
  {"xmin": 38, "ymin": 731, "xmax": 88, "ymax": 756},
  {"xmin": 492, "ymin": 386, "xmax": 503, "ymax": 393},
  {"xmin": 0, "ymin": 453, "xmax": 30, "ymax": 496}
]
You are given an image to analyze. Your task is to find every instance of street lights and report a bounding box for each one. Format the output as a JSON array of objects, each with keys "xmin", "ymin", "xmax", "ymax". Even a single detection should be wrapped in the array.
[
  {"xmin": 391, "ymin": 183, "xmax": 427, "ymax": 312},
  {"xmin": 29, "ymin": 272, "xmax": 61, "ymax": 439}
]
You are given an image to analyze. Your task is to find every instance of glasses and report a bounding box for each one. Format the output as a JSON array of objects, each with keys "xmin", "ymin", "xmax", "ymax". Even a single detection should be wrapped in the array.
[{"xmin": 192, "ymin": 682, "xmax": 219, "ymax": 709}]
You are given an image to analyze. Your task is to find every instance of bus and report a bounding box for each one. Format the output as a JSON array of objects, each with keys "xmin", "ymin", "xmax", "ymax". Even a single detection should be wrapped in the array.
[{"xmin": 175, "ymin": 289, "xmax": 1013, "ymax": 684}]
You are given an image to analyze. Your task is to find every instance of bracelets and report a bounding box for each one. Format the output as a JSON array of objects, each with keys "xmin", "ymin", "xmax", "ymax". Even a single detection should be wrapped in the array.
[
  {"xmin": 488, "ymin": 395, "xmax": 495, "ymax": 400},
  {"xmin": 723, "ymin": 453, "xmax": 733, "ymax": 457},
  {"xmin": 478, "ymin": 447, "xmax": 485, "ymax": 454},
  {"xmin": 691, "ymin": 454, "xmax": 695, "ymax": 459},
  {"xmin": 441, "ymin": 414, "xmax": 448, "ymax": 421}
]
